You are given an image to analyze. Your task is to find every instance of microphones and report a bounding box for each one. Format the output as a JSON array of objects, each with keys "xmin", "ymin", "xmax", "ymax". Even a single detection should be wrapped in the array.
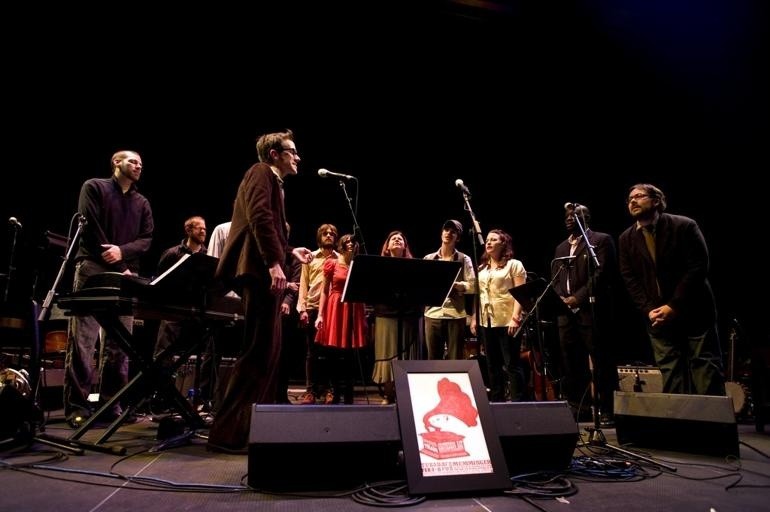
[
  {"xmin": 8, "ymin": 216, "xmax": 23, "ymax": 231},
  {"xmin": 555, "ymin": 255, "xmax": 576, "ymax": 260},
  {"xmin": 564, "ymin": 202, "xmax": 576, "ymax": 210},
  {"xmin": 455, "ymin": 179, "xmax": 472, "ymax": 196},
  {"xmin": 318, "ymin": 168, "xmax": 353, "ymax": 180}
]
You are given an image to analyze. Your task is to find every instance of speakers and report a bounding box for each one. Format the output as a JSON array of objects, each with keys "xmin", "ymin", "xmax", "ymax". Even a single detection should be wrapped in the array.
[
  {"xmin": 248, "ymin": 404, "xmax": 402, "ymax": 494},
  {"xmin": 489, "ymin": 401, "xmax": 579, "ymax": 475},
  {"xmin": 613, "ymin": 391, "xmax": 741, "ymax": 460}
]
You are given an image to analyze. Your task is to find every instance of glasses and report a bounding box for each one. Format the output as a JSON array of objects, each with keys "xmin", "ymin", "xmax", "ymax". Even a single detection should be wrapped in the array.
[
  {"xmin": 626, "ymin": 193, "xmax": 649, "ymax": 204},
  {"xmin": 279, "ymin": 147, "xmax": 297, "ymax": 155}
]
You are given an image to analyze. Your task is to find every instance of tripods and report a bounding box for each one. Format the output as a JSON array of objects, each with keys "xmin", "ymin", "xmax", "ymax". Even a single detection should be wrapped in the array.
[
  {"xmin": 571, "ymin": 209, "xmax": 677, "ymax": 472},
  {"xmin": 0, "ymin": 223, "xmax": 85, "ymax": 455}
]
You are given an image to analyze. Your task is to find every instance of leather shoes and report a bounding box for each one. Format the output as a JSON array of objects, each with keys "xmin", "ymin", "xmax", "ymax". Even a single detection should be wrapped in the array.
[
  {"xmin": 69, "ymin": 417, "xmax": 86, "ymax": 427},
  {"xmin": 113, "ymin": 415, "xmax": 137, "ymax": 422}
]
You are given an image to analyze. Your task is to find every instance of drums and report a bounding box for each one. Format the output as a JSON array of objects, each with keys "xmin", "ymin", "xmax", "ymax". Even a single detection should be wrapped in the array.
[{"xmin": 724, "ymin": 381, "xmax": 751, "ymax": 417}]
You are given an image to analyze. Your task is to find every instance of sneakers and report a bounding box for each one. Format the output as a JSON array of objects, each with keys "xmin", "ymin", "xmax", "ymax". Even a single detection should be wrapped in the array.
[
  {"xmin": 302, "ymin": 394, "xmax": 315, "ymax": 404},
  {"xmin": 325, "ymin": 393, "xmax": 335, "ymax": 404}
]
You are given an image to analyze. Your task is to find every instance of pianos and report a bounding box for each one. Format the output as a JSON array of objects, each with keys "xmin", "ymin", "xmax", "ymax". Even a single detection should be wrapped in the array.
[{"xmin": 54, "ymin": 272, "xmax": 247, "ymax": 322}]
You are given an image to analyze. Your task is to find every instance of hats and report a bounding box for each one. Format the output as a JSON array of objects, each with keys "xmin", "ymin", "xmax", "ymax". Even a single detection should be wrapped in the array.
[{"xmin": 442, "ymin": 219, "xmax": 463, "ymax": 234}]
